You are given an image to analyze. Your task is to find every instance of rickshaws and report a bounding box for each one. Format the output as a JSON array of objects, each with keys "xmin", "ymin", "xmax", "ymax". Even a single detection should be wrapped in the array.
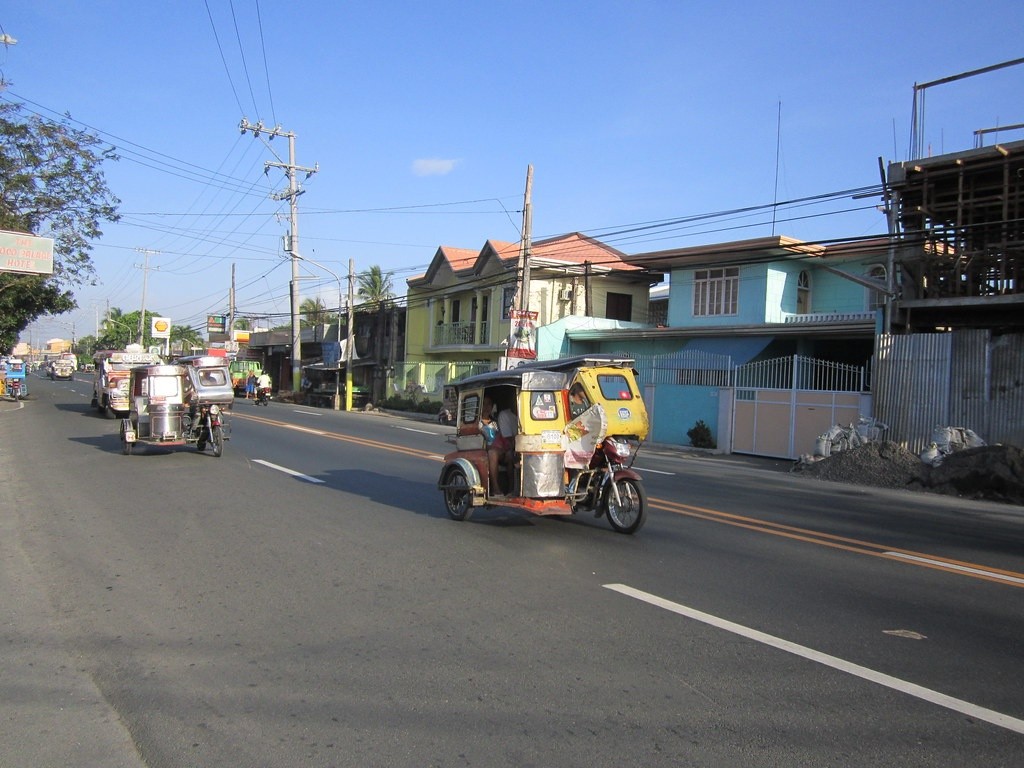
[
  {"xmin": 0, "ymin": 356, "xmax": 31, "ymax": 401},
  {"xmin": 437, "ymin": 354, "xmax": 649, "ymax": 534},
  {"xmin": 120, "ymin": 357, "xmax": 234, "ymax": 457}
]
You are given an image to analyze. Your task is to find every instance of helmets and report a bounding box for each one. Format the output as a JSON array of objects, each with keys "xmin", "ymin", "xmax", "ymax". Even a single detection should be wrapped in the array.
[{"xmin": 262, "ymin": 370, "xmax": 266, "ymax": 373}]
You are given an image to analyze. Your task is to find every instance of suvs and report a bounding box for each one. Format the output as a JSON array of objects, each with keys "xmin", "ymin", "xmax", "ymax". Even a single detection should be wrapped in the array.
[{"xmin": 230, "ymin": 361, "xmax": 272, "ymax": 397}]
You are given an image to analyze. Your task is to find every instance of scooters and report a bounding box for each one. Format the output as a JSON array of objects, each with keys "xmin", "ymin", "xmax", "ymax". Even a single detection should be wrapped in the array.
[{"xmin": 253, "ymin": 382, "xmax": 271, "ymax": 406}]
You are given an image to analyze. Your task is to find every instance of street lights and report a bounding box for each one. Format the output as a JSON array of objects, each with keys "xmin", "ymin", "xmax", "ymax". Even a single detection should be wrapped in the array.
[
  {"xmin": 290, "ymin": 251, "xmax": 340, "ymax": 410},
  {"xmin": 110, "ymin": 319, "xmax": 132, "ymax": 345}
]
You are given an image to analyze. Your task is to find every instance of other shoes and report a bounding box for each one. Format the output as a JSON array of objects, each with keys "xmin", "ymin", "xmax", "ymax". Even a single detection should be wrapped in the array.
[
  {"xmin": 253, "ymin": 398, "xmax": 258, "ymax": 400},
  {"xmin": 492, "ymin": 492, "xmax": 503, "ymax": 497},
  {"xmin": 245, "ymin": 398, "xmax": 248, "ymax": 399}
]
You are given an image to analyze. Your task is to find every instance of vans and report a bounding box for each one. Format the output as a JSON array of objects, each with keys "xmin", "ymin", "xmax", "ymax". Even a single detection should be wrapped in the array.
[
  {"xmin": 45, "ymin": 352, "xmax": 77, "ymax": 381},
  {"xmin": 91, "ymin": 350, "xmax": 165, "ymax": 413}
]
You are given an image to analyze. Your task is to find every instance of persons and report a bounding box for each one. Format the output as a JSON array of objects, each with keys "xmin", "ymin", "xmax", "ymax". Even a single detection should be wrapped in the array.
[
  {"xmin": 245, "ymin": 370, "xmax": 256, "ymax": 399},
  {"xmin": 253, "ymin": 370, "xmax": 270, "ymax": 401},
  {"xmin": 200, "ymin": 371, "xmax": 217, "ymax": 385},
  {"xmin": 564, "ymin": 384, "xmax": 589, "ymax": 494},
  {"xmin": 481, "ymin": 388, "xmax": 521, "ymax": 498}
]
